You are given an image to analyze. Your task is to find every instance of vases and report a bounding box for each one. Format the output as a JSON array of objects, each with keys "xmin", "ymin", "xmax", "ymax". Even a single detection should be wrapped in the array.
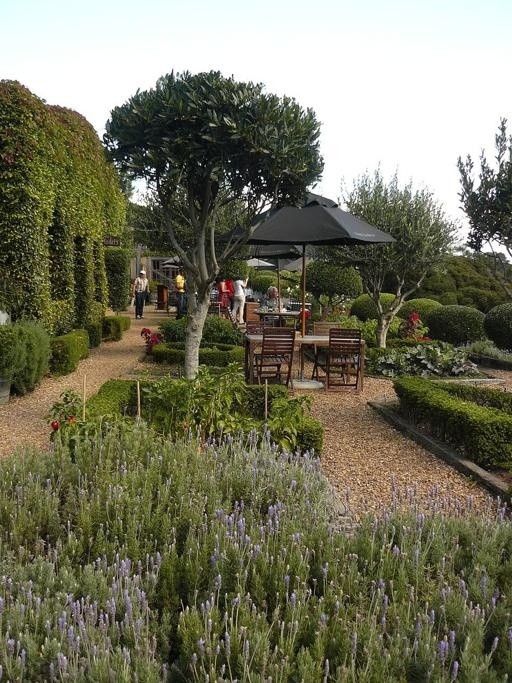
[{"xmin": 146, "ymin": 344, "xmax": 153, "ymax": 354}]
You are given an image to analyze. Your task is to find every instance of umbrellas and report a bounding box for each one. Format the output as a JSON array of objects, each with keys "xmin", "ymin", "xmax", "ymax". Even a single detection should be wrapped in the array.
[
  {"xmin": 161, "ymin": 254, "xmax": 183, "ymax": 266},
  {"xmin": 240, "ymin": 238, "xmax": 312, "ymax": 327},
  {"xmin": 246, "ymin": 258, "xmax": 276, "ymax": 268},
  {"xmin": 246, "ymin": 192, "xmax": 399, "ymax": 380}
]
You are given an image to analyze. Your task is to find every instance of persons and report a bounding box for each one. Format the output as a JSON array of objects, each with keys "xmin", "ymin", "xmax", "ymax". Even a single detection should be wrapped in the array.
[
  {"xmin": 231, "ymin": 276, "xmax": 247, "ymax": 323},
  {"xmin": 217, "ymin": 278, "xmax": 231, "ymax": 309},
  {"xmin": 175, "ymin": 269, "xmax": 185, "ymax": 320},
  {"xmin": 132, "ymin": 270, "xmax": 151, "ymax": 319},
  {"xmin": 263, "ymin": 286, "xmax": 284, "ymax": 325}
]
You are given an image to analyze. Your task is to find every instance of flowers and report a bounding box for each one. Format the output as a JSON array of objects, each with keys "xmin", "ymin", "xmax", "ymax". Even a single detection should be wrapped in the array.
[{"xmin": 140, "ymin": 326, "xmax": 162, "ymax": 344}]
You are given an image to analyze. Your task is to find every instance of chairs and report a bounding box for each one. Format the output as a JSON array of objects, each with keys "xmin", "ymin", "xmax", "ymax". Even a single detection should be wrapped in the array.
[{"xmin": 244, "ymin": 302, "xmax": 366, "ymax": 393}]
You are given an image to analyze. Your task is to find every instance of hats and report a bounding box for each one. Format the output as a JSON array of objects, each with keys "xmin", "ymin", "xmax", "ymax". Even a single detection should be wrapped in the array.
[{"xmin": 140, "ymin": 270, "xmax": 146, "ymax": 274}]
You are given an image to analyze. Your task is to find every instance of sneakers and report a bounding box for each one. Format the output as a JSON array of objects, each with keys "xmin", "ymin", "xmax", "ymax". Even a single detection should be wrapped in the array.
[{"xmin": 136, "ymin": 315, "xmax": 143, "ymax": 319}]
